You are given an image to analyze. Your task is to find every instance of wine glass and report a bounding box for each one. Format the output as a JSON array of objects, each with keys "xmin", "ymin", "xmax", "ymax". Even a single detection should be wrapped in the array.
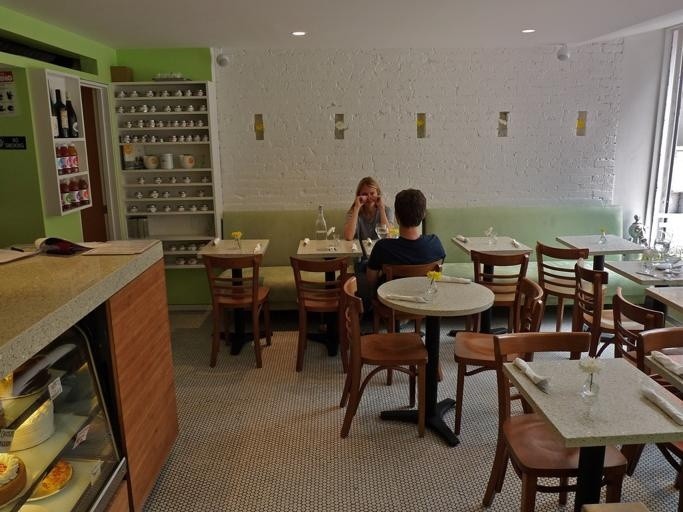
[{"xmin": 636, "ymin": 230, "xmax": 682, "ymax": 280}]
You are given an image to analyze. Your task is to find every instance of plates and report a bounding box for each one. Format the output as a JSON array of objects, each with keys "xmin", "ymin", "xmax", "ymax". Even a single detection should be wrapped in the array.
[{"xmin": 26, "ymin": 461, "xmax": 72, "ymax": 503}]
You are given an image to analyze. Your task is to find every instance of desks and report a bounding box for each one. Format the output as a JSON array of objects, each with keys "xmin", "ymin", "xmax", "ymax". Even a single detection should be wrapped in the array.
[
  {"xmin": 203, "ymin": 238, "xmax": 274, "ymax": 357},
  {"xmin": 448, "ymin": 235, "xmax": 533, "ymax": 340},
  {"xmin": 558, "ymin": 235, "xmax": 648, "ymax": 344},
  {"xmin": 362, "ymin": 238, "xmax": 381, "ymax": 260},
  {"xmin": 646, "ymin": 353, "xmax": 682, "ymax": 392},
  {"xmin": 645, "ymin": 287, "xmax": 683, "ymax": 314},
  {"xmin": 603, "ymin": 260, "xmax": 683, "ymax": 326},
  {"xmin": 503, "ymin": 358, "xmax": 682, "ymax": 508},
  {"xmin": 298, "ymin": 238, "xmax": 364, "ymax": 357}
]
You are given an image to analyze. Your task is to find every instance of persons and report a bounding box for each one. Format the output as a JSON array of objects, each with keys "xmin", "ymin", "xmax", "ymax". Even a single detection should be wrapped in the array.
[
  {"xmin": 319, "ymin": 187, "xmax": 448, "ymax": 334},
  {"xmin": 342, "ymin": 176, "xmax": 395, "ymax": 242}
]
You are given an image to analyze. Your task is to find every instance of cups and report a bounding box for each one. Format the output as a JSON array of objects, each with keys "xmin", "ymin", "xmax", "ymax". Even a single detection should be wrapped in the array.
[
  {"xmin": 143, "ymin": 154, "xmax": 159, "ymax": 169},
  {"xmin": 374, "ymin": 222, "xmax": 388, "ymax": 239},
  {"xmin": 488, "ymin": 233, "xmax": 497, "ymax": 247},
  {"xmin": 179, "ymin": 154, "xmax": 195, "ymax": 168},
  {"xmin": 159, "ymin": 153, "xmax": 173, "ymax": 169}
]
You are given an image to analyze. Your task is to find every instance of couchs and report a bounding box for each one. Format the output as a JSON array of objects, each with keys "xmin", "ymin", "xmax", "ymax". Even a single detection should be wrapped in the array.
[
  {"xmin": 226, "ymin": 207, "xmax": 392, "ymax": 312},
  {"xmin": 426, "ymin": 202, "xmax": 660, "ymax": 306}
]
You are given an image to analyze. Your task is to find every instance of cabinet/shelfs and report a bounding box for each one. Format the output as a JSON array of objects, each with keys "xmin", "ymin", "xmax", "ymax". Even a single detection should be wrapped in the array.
[
  {"xmin": 110, "ymin": 74, "xmax": 224, "ymax": 273},
  {"xmin": 26, "ymin": 64, "xmax": 91, "ymax": 220},
  {"xmin": 0, "ymin": 324, "xmax": 132, "ymax": 511}
]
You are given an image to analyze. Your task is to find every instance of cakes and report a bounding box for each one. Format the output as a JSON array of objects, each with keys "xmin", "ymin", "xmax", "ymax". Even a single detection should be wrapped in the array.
[
  {"xmin": 0, "ymin": 346, "xmax": 52, "ymax": 395},
  {"xmin": 8, "ymin": 399, "xmax": 55, "ymax": 452},
  {"xmin": 31, "ymin": 459, "xmax": 73, "ymax": 498},
  {"xmin": 0, "ymin": 453, "xmax": 27, "ymax": 506}
]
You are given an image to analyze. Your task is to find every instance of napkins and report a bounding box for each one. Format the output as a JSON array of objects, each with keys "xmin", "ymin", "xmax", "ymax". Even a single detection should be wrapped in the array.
[
  {"xmin": 252, "ymin": 242, "xmax": 262, "ymax": 254},
  {"xmin": 511, "ymin": 238, "xmax": 520, "ymax": 249},
  {"xmin": 213, "ymin": 237, "xmax": 220, "ymax": 246},
  {"xmin": 513, "ymin": 357, "xmax": 552, "ymax": 395},
  {"xmin": 642, "ymin": 386, "xmax": 682, "ymax": 426},
  {"xmin": 442, "ymin": 275, "xmax": 471, "ymax": 283},
  {"xmin": 367, "ymin": 237, "xmax": 373, "ymax": 246},
  {"xmin": 387, "ymin": 294, "xmax": 425, "ymax": 304},
  {"xmin": 650, "ymin": 351, "xmax": 682, "ymax": 376},
  {"xmin": 303, "ymin": 237, "xmax": 310, "ymax": 246},
  {"xmin": 352, "ymin": 243, "xmax": 358, "ymax": 251},
  {"xmin": 456, "ymin": 234, "xmax": 469, "ymax": 243}
]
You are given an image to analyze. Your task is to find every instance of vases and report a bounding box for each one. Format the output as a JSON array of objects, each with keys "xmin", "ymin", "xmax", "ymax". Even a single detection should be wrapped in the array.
[
  {"xmin": 584, "ymin": 376, "xmax": 599, "ymax": 399},
  {"xmin": 598, "ymin": 234, "xmax": 607, "ymax": 246},
  {"xmin": 489, "ymin": 231, "xmax": 498, "ymax": 246},
  {"xmin": 429, "ymin": 280, "xmax": 440, "ymax": 296},
  {"xmin": 645, "ymin": 263, "xmax": 656, "ymax": 277},
  {"xmin": 334, "ymin": 234, "xmax": 342, "ymax": 250},
  {"xmin": 233, "ymin": 241, "xmax": 242, "ymax": 251}
]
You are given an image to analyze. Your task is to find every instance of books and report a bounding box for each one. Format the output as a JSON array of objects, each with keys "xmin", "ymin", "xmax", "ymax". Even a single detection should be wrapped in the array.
[{"xmin": 0, "ymin": 230, "xmax": 112, "ymax": 266}]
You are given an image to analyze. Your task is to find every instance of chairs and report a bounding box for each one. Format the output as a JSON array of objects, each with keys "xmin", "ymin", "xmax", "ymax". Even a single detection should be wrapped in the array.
[
  {"xmin": 205, "ymin": 256, "xmax": 271, "ymax": 370},
  {"xmin": 614, "ymin": 285, "xmax": 665, "ymax": 369},
  {"xmin": 482, "ymin": 330, "xmax": 627, "ymax": 508},
  {"xmin": 291, "ymin": 256, "xmax": 354, "ymax": 374},
  {"xmin": 572, "ymin": 263, "xmax": 645, "ymax": 362},
  {"xmin": 455, "ymin": 278, "xmax": 545, "ymax": 436},
  {"xmin": 338, "ymin": 276, "xmax": 427, "ymax": 439},
  {"xmin": 620, "ymin": 327, "xmax": 683, "ymax": 490},
  {"xmin": 536, "ymin": 241, "xmax": 589, "ymax": 332},
  {"xmin": 472, "ymin": 248, "xmax": 529, "ymax": 334},
  {"xmin": 374, "ymin": 258, "xmax": 445, "ymax": 385}
]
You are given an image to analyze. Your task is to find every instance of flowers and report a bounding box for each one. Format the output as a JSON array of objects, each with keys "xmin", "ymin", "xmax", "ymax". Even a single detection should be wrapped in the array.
[
  {"xmin": 389, "ymin": 224, "xmax": 399, "ymax": 239},
  {"xmin": 642, "ymin": 249, "xmax": 657, "ymax": 263},
  {"xmin": 485, "ymin": 226, "xmax": 494, "ymax": 238},
  {"xmin": 600, "ymin": 225, "xmax": 608, "ymax": 234},
  {"xmin": 575, "ymin": 355, "xmax": 605, "ymax": 379},
  {"xmin": 327, "ymin": 225, "xmax": 338, "ymax": 238},
  {"xmin": 427, "ymin": 271, "xmax": 442, "ymax": 282},
  {"xmin": 231, "ymin": 231, "xmax": 243, "ymax": 240}
]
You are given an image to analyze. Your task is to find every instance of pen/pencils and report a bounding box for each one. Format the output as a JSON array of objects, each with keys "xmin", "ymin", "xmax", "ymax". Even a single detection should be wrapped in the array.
[{"xmin": 11, "ymin": 246, "xmax": 25, "ymax": 253}]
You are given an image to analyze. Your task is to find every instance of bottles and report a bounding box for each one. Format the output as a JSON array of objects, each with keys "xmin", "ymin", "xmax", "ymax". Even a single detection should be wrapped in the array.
[
  {"xmin": 313, "ymin": 204, "xmax": 328, "ymax": 253},
  {"xmin": 48, "ymin": 89, "xmax": 78, "ymax": 138},
  {"xmin": 54, "ymin": 144, "xmax": 78, "ymax": 176},
  {"xmin": 126, "ymin": 217, "xmax": 149, "ymax": 239},
  {"xmin": 597, "ymin": 230, "xmax": 607, "ymax": 245},
  {"xmin": 59, "ymin": 176, "xmax": 90, "ymax": 213}
]
